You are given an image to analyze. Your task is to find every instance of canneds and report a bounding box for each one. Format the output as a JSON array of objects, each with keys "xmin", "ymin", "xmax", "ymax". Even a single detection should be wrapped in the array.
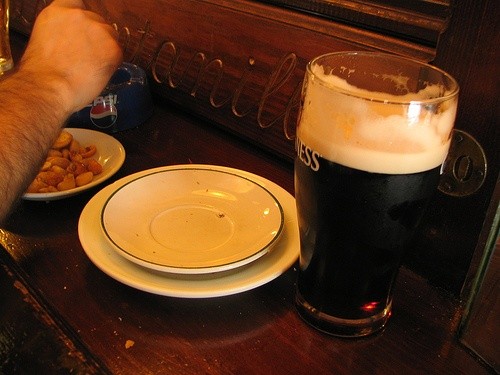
[{"xmin": 76, "ymin": 83, "xmax": 120, "ymax": 141}]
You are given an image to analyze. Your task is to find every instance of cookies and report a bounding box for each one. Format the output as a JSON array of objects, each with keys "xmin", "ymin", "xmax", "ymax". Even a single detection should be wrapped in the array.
[{"xmin": 28, "ymin": 131, "xmax": 102, "ymax": 193}]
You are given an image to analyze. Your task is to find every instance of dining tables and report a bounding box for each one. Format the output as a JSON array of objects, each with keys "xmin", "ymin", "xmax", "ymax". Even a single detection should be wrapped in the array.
[{"xmin": 0, "ymin": 107, "xmax": 500, "ymax": 375}]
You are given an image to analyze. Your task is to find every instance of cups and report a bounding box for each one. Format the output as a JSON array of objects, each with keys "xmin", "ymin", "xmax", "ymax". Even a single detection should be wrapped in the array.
[
  {"xmin": 0, "ymin": 0, "xmax": 15, "ymax": 77},
  {"xmin": 293, "ymin": 50, "xmax": 460, "ymax": 338}
]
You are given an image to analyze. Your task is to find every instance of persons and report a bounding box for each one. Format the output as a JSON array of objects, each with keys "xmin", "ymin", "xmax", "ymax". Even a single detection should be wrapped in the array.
[{"xmin": 0, "ymin": 0, "xmax": 124, "ymax": 235}]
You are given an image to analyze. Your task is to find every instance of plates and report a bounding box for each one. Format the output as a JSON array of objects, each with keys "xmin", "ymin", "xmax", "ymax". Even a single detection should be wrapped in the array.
[
  {"xmin": 21, "ymin": 127, "xmax": 126, "ymax": 200},
  {"xmin": 100, "ymin": 168, "xmax": 285, "ymax": 275},
  {"xmin": 78, "ymin": 164, "xmax": 301, "ymax": 298}
]
